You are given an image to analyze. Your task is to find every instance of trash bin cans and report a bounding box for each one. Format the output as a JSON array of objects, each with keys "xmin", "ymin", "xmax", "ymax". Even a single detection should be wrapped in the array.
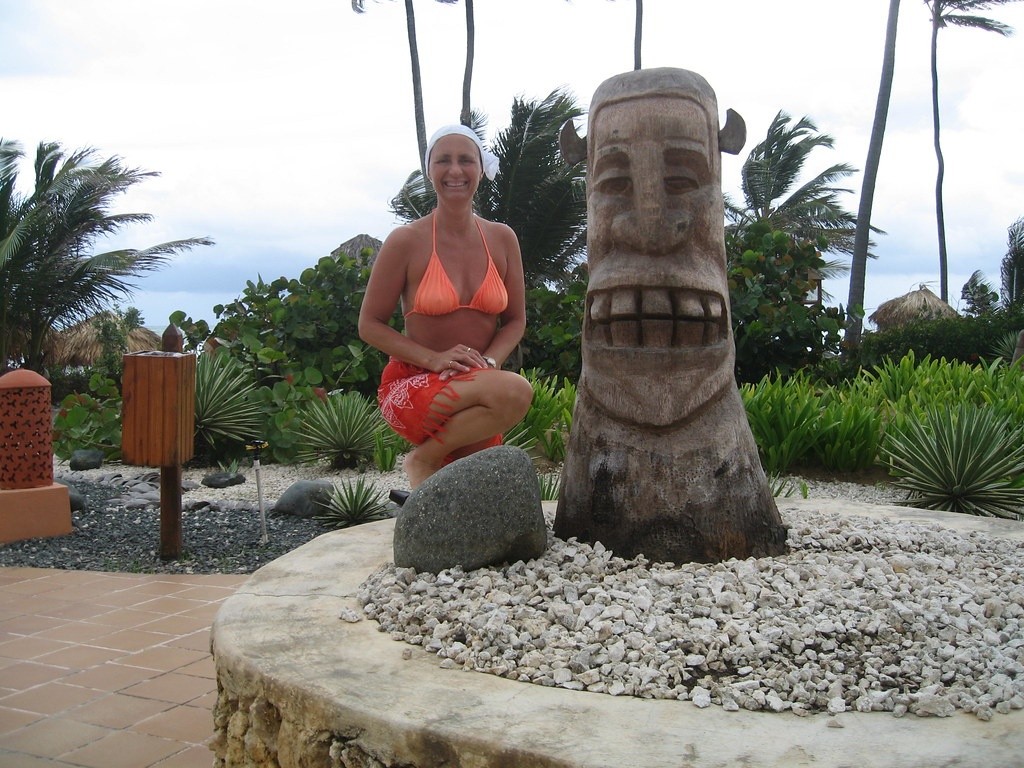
[{"xmin": 122, "ymin": 350, "xmax": 197, "ymax": 468}]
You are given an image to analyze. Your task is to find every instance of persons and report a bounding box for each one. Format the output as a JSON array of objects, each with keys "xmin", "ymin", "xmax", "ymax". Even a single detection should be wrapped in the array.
[{"xmin": 358, "ymin": 125, "xmax": 533, "ymax": 489}]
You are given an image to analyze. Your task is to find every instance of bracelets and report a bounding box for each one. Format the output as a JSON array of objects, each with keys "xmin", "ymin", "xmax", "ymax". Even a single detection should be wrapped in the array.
[{"xmin": 482, "ymin": 355, "xmax": 496, "ymax": 367}]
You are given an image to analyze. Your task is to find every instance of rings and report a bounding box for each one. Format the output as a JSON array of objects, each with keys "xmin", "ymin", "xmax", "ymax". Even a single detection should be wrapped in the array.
[
  {"xmin": 467, "ymin": 348, "xmax": 470, "ymax": 352},
  {"xmin": 449, "ymin": 361, "xmax": 453, "ymax": 368}
]
